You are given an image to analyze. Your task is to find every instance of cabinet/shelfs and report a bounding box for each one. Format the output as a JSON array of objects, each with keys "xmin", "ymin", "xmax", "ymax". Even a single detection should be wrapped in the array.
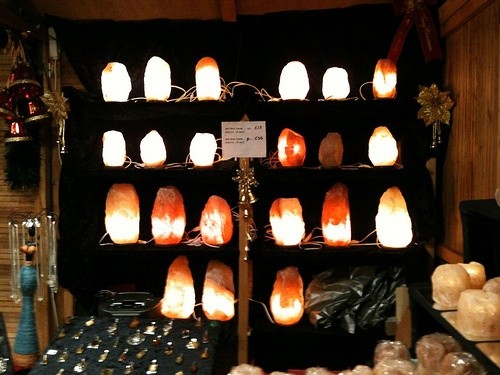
[{"xmin": 28, "ymin": 7, "xmax": 500, "ymax": 375}]
